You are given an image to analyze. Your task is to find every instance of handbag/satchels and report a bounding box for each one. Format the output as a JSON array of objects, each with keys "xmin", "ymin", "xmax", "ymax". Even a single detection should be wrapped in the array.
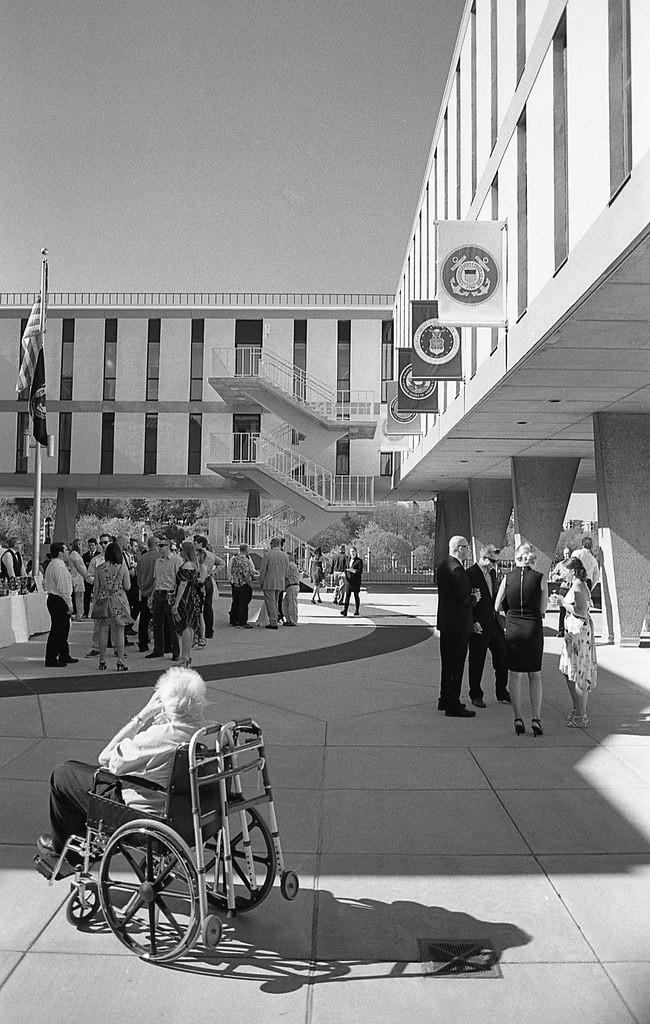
[{"xmin": 91, "ymin": 598, "xmax": 110, "ymax": 618}]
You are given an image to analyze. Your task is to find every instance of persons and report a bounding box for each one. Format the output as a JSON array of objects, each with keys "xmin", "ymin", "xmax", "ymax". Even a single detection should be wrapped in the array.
[
  {"xmin": 572, "ymin": 537, "xmax": 600, "ymax": 594},
  {"xmin": 552, "ymin": 547, "xmax": 573, "ymax": 637},
  {"xmin": 437, "ymin": 534, "xmax": 481, "ymax": 717},
  {"xmin": 227, "ymin": 542, "xmax": 258, "ymax": 628},
  {"xmin": 309, "ymin": 546, "xmax": 326, "ymax": 604},
  {"xmin": 44, "ymin": 533, "xmax": 226, "ymax": 671},
  {"xmin": 37, "ymin": 667, "xmax": 207, "ymax": 872},
  {"xmin": 259, "ymin": 537, "xmax": 300, "ymax": 628},
  {"xmin": 0, "ymin": 536, "xmax": 44, "ymax": 578},
  {"xmin": 466, "ymin": 544, "xmax": 511, "ymax": 708},
  {"xmin": 549, "ymin": 557, "xmax": 598, "ymax": 729},
  {"xmin": 494, "ymin": 542, "xmax": 548, "ymax": 737},
  {"xmin": 340, "ymin": 547, "xmax": 363, "ymax": 617},
  {"xmin": 329, "ymin": 545, "xmax": 348, "ymax": 605}
]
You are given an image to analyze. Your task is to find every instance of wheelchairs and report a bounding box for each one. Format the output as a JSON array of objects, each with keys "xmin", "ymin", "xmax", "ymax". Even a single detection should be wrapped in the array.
[{"xmin": 31, "ymin": 716, "xmax": 299, "ymax": 966}]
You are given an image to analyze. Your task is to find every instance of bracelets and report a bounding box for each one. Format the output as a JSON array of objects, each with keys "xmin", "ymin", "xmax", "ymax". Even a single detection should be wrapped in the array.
[{"xmin": 134, "ymin": 716, "xmax": 144, "ymax": 727}]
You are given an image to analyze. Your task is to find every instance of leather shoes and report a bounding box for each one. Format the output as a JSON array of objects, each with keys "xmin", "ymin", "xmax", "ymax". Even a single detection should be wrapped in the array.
[{"xmin": 37, "ymin": 834, "xmax": 85, "ymax": 871}]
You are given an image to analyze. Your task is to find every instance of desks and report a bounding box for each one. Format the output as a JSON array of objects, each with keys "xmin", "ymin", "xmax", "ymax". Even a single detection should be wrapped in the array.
[{"xmin": 0, "ymin": 591, "xmax": 49, "ymax": 648}]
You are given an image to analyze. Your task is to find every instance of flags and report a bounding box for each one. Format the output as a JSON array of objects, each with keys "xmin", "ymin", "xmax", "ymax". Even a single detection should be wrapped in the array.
[
  {"xmin": 29, "ymin": 346, "xmax": 48, "ymax": 448},
  {"xmin": 15, "ymin": 262, "xmax": 48, "ymax": 394}
]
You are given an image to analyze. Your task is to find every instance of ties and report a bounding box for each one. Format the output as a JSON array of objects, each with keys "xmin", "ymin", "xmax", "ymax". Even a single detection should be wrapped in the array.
[{"xmin": 91, "ymin": 553, "xmax": 93, "ymax": 556}]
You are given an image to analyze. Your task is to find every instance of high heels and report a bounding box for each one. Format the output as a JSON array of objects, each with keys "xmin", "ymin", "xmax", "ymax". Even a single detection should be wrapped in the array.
[
  {"xmin": 531, "ymin": 718, "xmax": 544, "ymax": 738},
  {"xmin": 514, "ymin": 718, "xmax": 526, "ymax": 736},
  {"xmin": 565, "ymin": 708, "xmax": 589, "ymax": 728}
]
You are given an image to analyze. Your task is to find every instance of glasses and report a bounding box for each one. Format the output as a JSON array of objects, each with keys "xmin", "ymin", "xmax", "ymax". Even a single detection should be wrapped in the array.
[
  {"xmin": 99, "ymin": 540, "xmax": 108, "ymax": 545},
  {"xmin": 485, "ymin": 556, "xmax": 499, "ymax": 563},
  {"xmin": 457, "ymin": 544, "xmax": 470, "ymax": 550},
  {"xmin": 158, "ymin": 544, "xmax": 168, "ymax": 548}
]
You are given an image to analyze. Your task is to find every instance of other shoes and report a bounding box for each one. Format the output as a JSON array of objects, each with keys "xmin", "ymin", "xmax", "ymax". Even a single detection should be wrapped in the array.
[
  {"xmin": 435, "ymin": 690, "xmax": 512, "ymax": 718},
  {"xmin": 83, "ymin": 628, "xmax": 214, "ymax": 670},
  {"xmin": 58, "ymin": 657, "xmax": 79, "ymax": 664},
  {"xmin": 55, "ymin": 663, "xmax": 65, "ymax": 667},
  {"xmin": 265, "ymin": 624, "xmax": 278, "ymax": 629}
]
